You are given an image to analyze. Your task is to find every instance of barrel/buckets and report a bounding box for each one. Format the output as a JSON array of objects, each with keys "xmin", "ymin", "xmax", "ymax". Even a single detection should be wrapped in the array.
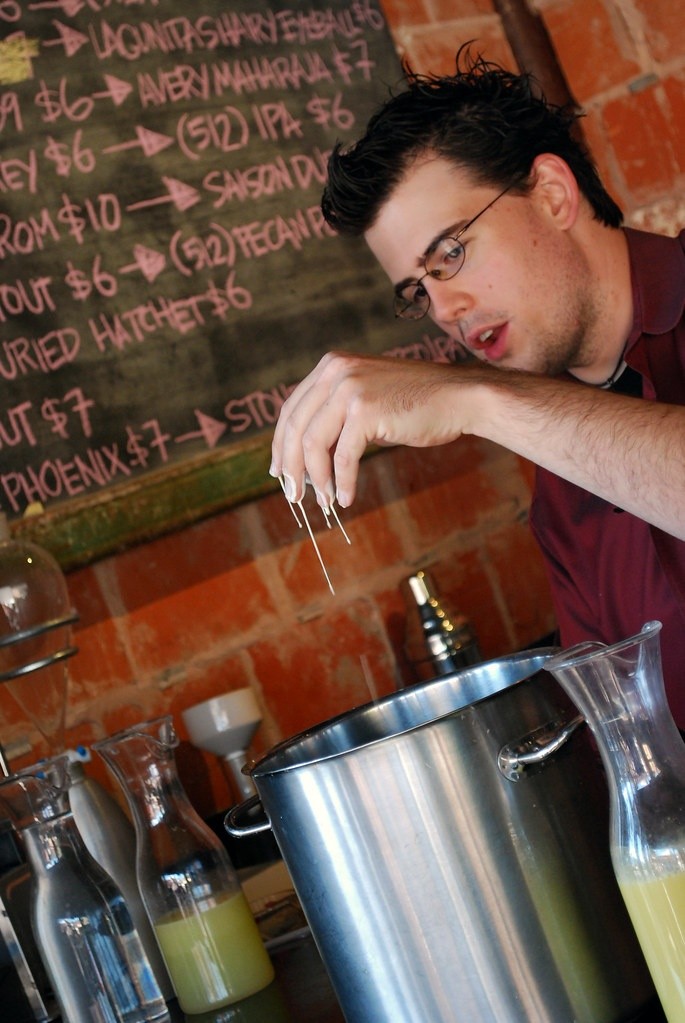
[{"xmin": 223, "ymin": 643, "xmax": 662, "ymax": 1023}]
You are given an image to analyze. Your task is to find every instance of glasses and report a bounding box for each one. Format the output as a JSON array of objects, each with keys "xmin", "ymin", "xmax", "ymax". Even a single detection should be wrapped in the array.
[{"xmin": 392, "ymin": 169, "xmax": 532, "ymax": 319}]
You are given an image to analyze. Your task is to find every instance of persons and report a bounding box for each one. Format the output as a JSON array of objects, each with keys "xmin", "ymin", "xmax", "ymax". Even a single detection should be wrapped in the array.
[{"xmin": 269, "ymin": 36, "xmax": 684, "ymax": 730}]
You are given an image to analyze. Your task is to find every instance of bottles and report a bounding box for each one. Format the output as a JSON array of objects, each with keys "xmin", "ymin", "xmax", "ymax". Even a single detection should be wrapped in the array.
[
  {"xmin": 544, "ymin": 618, "xmax": 685, "ymax": 1023},
  {"xmin": 395, "ymin": 571, "xmax": 484, "ymax": 678},
  {"xmin": 0, "ymin": 759, "xmax": 174, "ymax": 1023},
  {"xmin": 89, "ymin": 712, "xmax": 274, "ymax": 1016}
]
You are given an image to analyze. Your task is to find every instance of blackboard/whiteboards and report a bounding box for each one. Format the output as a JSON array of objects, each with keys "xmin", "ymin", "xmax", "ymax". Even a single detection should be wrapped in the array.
[{"xmin": 1, "ymin": 0, "xmax": 488, "ymax": 573}]
